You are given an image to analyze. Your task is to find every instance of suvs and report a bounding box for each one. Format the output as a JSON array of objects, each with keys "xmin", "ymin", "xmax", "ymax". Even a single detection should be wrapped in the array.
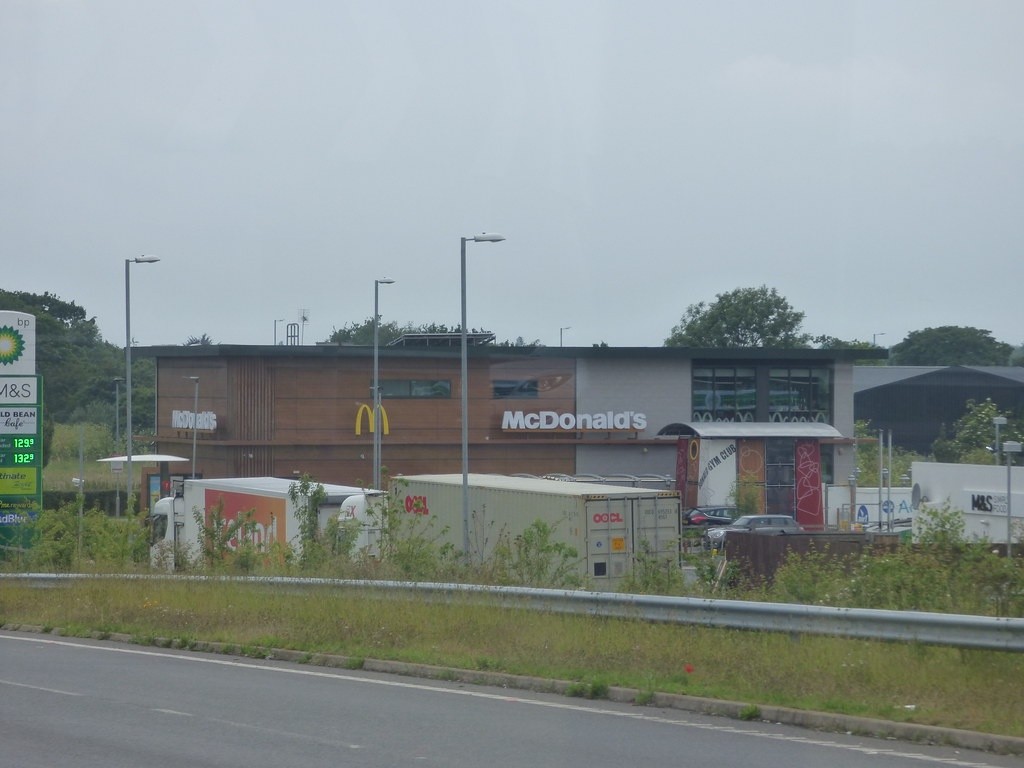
[
  {"xmin": 682, "ymin": 503, "xmax": 751, "ymax": 547},
  {"xmin": 700, "ymin": 514, "xmax": 807, "ymax": 552}
]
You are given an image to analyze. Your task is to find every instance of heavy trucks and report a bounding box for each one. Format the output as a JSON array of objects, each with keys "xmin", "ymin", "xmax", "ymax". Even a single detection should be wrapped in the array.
[
  {"xmin": 336, "ymin": 474, "xmax": 683, "ymax": 620},
  {"xmin": 145, "ymin": 473, "xmax": 390, "ymax": 578}
]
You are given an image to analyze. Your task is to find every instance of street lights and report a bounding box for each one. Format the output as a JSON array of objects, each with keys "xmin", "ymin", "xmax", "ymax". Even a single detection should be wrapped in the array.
[
  {"xmin": 122, "ymin": 254, "xmax": 162, "ymax": 545},
  {"xmin": 188, "ymin": 376, "xmax": 200, "ymax": 480},
  {"xmin": 273, "ymin": 318, "xmax": 284, "ymax": 344},
  {"xmin": 1003, "ymin": 440, "xmax": 1022, "ymax": 557},
  {"xmin": 372, "ymin": 276, "xmax": 396, "ymax": 491},
  {"xmin": 994, "ymin": 416, "xmax": 1008, "ymax": 466},
  {"xmin": 458, "ymin": 232, "xmax": 507, "ymax": 574},
  {"xmin": 112, "ymin": 377, "xmax": 127, "ymax": 518},
  {"xmin": 560, "ymin": 326, "xmax": 571, "ymax": 347},
  {"xmin": 873, "ymin": 332, "xmax": 885, "ymax": 347}
]
